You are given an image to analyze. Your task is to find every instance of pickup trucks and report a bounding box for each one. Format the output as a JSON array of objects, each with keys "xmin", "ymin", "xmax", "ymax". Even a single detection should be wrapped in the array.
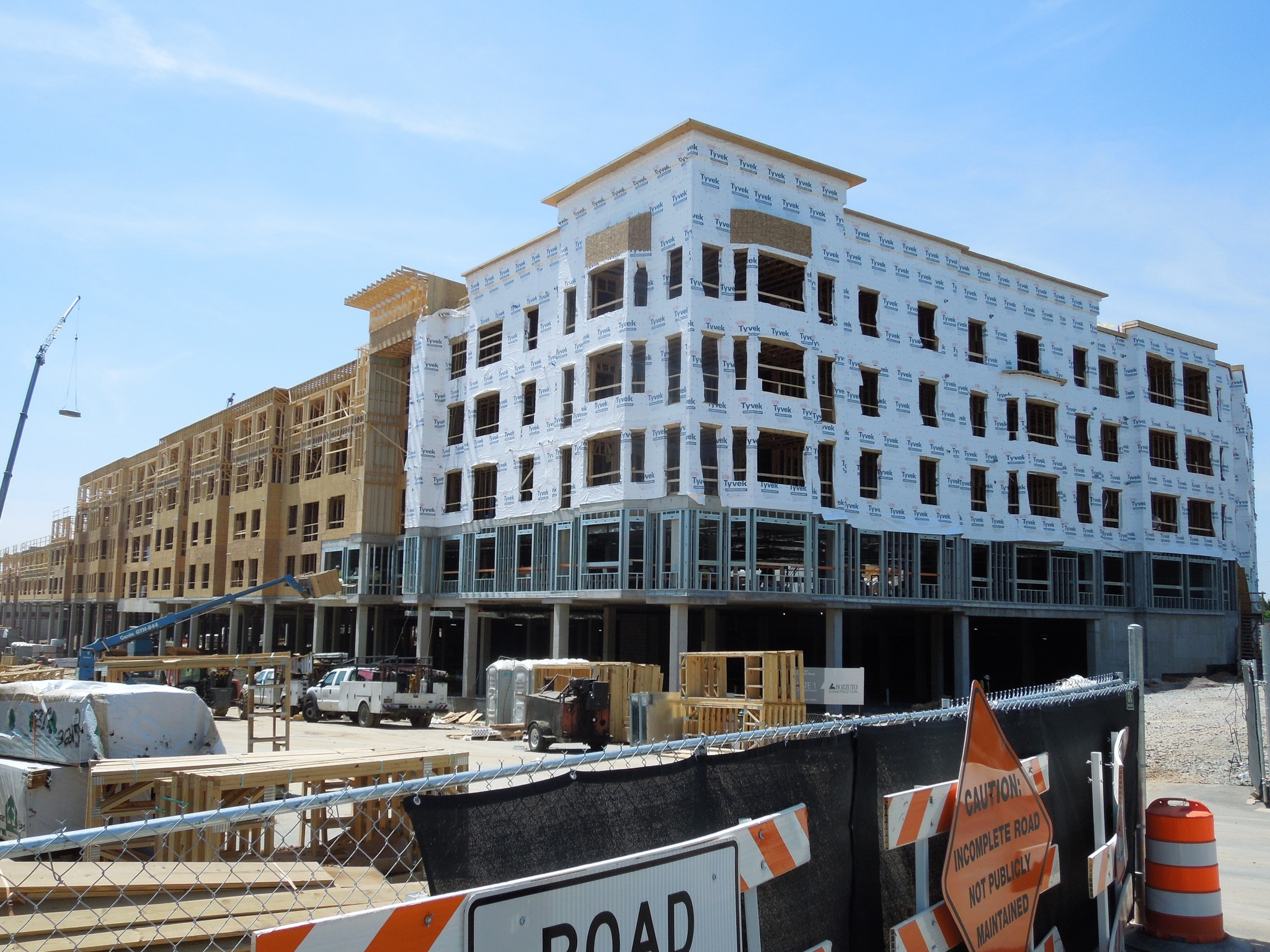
[{"xmin": 241, "ymin": 666, "xmax": 448, "ymax": 728}]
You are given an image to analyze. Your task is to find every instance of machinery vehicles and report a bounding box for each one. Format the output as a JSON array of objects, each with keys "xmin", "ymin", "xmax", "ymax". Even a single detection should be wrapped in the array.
[
  {"xmin": 80, "ymin": 565, "xmax": 341, "ymax": 683},
  {"xmin": 164, "ymin": 647, "xmax": 251, "ymax": 720},
  {"xmin": 0, "ymin": 294, "xmax": 82, "ymax": 515}
]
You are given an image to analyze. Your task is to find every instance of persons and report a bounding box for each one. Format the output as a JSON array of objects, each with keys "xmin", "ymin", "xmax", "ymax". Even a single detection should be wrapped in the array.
[
  {"xmin": 605, "ymin": 446, "xmax": 612, "ymax": 468},
  {"xmin": 335, "ymin": 565, "xmax": 381, "ymax": 595},
  {"xmin": 700, "ymin": 566, "xmax": 709, "ymax": 577},
  {"xmin": 1152, "ymin": 514, "xmax": 1162, "ymax": 531}
]
